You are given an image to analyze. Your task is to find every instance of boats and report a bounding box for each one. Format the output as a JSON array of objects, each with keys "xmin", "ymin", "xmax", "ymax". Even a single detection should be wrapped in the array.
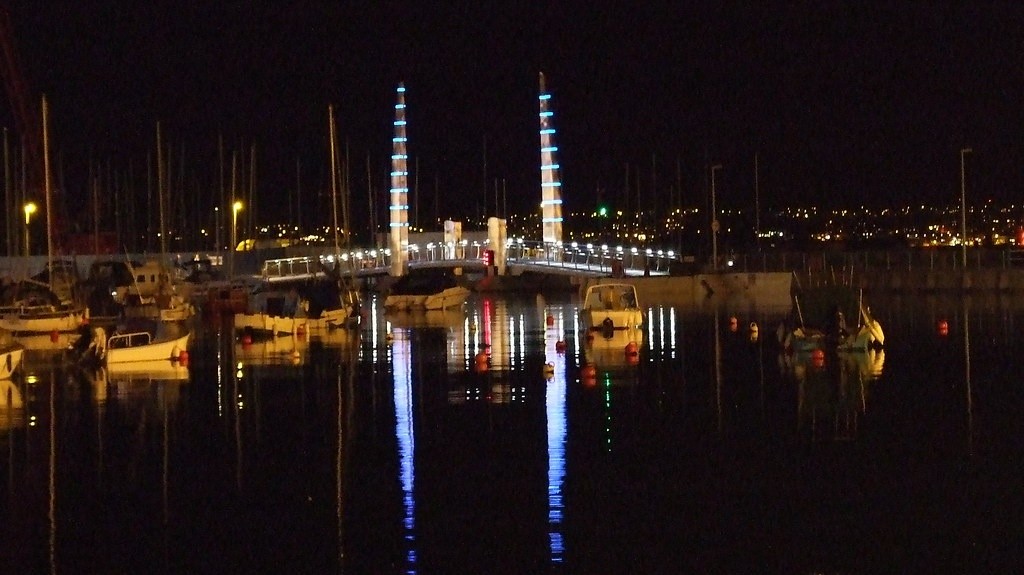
[{"xmin": 0, "ymin": 92, "xmax": 892, "ymax": 436}]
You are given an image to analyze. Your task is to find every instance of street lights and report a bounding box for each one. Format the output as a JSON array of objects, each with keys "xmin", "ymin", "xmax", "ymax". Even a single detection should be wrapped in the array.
[{"xmin": 960, "ymin": 147, "xmax": 972, "ymax": 249}]
